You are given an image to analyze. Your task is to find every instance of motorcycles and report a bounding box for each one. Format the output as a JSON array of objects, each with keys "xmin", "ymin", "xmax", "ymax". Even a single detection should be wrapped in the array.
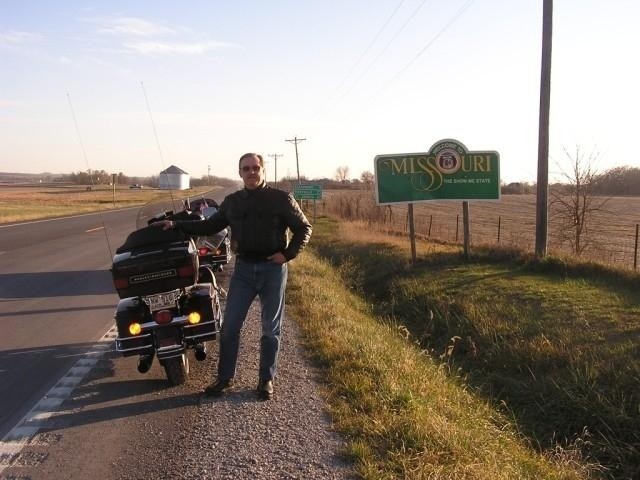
[{"xmin": 111, "ymin": 194, "xmax": 231, "ymax": 386}]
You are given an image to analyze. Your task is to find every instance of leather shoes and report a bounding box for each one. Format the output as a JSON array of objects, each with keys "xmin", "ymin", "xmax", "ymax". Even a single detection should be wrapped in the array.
[
  {"xmin": 257, "ymin": 378, "xmax": 274, "ymax": 400},
  {"xmin": 206, "ymin": 378, "xmax": 235, "ymax": 396}
]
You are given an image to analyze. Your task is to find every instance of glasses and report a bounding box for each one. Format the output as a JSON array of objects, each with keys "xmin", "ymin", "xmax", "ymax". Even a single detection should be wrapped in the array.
[{"xmin": 240, "ymin": 165, "xmax": 262, "ymax": 173}]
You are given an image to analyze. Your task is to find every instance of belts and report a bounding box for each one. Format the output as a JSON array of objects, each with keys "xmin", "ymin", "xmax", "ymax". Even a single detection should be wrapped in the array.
[{"xmin": 239, "ymin": 256, "xmax": 269, "ymax": 264}]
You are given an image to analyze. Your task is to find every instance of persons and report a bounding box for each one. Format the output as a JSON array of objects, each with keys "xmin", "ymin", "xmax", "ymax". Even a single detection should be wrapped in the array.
[{"xmin": 150, "ymin": 152, "xmax": 312, "ymax": 400}]
